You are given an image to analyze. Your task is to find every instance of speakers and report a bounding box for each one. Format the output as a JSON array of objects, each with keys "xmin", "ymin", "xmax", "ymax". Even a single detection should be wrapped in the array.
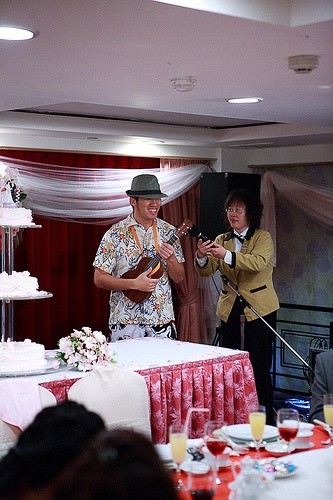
[{"xmin": 199, "ymin": 172, "xmax": 261, "ymax": 241}]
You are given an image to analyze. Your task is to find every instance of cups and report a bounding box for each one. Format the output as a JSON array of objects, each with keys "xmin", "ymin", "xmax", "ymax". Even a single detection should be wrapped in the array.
[
  {"xmin": 296, "ymin": 430, "xmax": 314, "ymax": 446},
  {"xmin": 187, "ymin": 462, "xmax": 215, "ymax": 500}
]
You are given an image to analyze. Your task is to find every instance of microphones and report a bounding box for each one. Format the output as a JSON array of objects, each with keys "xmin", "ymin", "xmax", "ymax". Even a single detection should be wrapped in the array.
[{"xmin": 189, "ymin": 224, "xmax": 216, "ymax": 248}]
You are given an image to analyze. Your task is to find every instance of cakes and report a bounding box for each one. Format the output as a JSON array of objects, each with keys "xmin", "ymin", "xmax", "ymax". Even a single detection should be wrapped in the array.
[
  {"xmin": 0, "ymin": 208, "xmax": 33, "ymax": 226},
  {"xmin": 0, "ymin": 338, "xmax": 46, "ymax": 373},
  {"xmin": 0, "ymin": 270, "xmax": 40, "ymax": 299}
]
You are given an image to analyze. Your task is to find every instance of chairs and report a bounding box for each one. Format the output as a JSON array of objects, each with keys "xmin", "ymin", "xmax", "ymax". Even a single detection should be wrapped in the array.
[{"xmin": 0, "ymin": 374, "xmax": 151, "ymax": 458}]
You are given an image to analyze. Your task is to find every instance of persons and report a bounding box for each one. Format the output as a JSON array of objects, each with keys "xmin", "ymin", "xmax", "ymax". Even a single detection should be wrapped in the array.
[
  {"xmin": 308, "ymin": 350, "xmax": 333, "ymax": 426},
  {"xmin": 193, "ymin": 188, "xmax": 280, "ymax": 427},
  {"xmin": 92, "ymin": 175, "xmax": 186, "ymax": 344},
  {"xmin": 0, "ymin": 400, "xmax": 177, "ymax": 500}
]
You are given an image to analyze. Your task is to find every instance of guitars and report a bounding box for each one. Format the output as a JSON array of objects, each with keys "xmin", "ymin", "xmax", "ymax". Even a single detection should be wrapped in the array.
[{"xmin": 117, "ymin": 219, "xmax": 195, "ymax": 304}]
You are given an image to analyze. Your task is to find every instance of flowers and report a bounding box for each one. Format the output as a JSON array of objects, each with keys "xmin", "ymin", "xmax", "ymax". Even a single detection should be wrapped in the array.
[{"xmin": 60, "ymin": 325, "xmax": 115, "ymax": 374}]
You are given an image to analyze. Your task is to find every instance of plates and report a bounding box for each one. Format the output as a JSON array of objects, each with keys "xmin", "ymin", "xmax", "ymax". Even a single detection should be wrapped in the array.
[
  {"xmin": 283, "ymin": 420, "xmax": 314, "ymax": 431},
  {"xmin": 264, "ymin": 443, "xmax": 291, "ymax": 457},
  {"xmin": 293, "ymin": 442, "xmax": 313, "ymax": 450},
  {"xmin": 222, "ymin": 422, "xmax": 282, "ymax": 446},
  {"xmin": 154, "ymin": 443, "xmax": 174, "ymax": 463},
  {"xmin": 179, "ymin": 459, "xmax": 210, "ymax": 477}
]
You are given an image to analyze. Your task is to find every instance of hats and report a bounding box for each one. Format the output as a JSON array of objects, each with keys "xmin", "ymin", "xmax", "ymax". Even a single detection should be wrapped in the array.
[{"xmin": 126, "ymin": 174, "xmax": 168, "ymax": 198}]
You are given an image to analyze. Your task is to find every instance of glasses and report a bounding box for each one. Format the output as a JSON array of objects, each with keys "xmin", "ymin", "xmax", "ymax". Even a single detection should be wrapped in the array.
[{"xmin": 226, "ymin": 207, "xmax": 247, "ymax": 214}]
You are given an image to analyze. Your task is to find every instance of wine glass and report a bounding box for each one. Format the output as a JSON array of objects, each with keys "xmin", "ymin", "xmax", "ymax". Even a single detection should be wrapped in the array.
[
  {"xmin": 276, "ymin": 409, "xmax": 300, "ymax": 459},
  {"xmin": 247, "ymin": 405, "xmax": 266, "ymax": 462},
  {"xmin": 205, "ymin": 420, "xmax": 227, "ymax": 485},
  {"xmin": 321, "ymin": 392, "xmax": 333, "ymax": 447},
  {"xmin": 168, "ymin": 425, "xmax": 189, "ymax": 492}
]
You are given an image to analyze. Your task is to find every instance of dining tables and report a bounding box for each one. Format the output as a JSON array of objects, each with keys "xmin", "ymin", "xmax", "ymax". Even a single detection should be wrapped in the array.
[
  {"xmin": 156, "ymin": 425, "xmax": 333, "ymax": 499},
  {"xmin": 0, "ymin": 336, "xmax": 260, "ymax": 460}
]
